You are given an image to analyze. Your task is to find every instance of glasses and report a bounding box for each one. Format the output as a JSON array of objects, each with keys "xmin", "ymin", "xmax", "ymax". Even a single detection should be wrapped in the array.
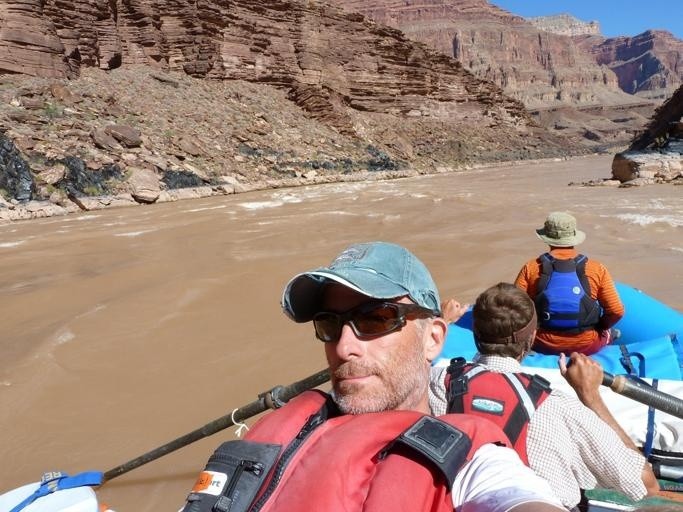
[{"xmin": 309, "ymin": 299, "xmax": 434, "ymax": 345}]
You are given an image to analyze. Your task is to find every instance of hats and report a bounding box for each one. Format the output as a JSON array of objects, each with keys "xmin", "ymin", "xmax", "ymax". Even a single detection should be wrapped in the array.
[
  {"xmin": 279, "ymin": 241, "xmax": 443, "ymax": 321},
  {"xmin": 535, "ymin": 211, "xmax": 586, "ymax": 247}
]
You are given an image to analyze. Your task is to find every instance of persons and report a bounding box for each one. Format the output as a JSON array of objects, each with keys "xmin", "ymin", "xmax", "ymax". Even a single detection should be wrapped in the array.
[
  {"xmin": 418, "ymin": 280, "xmax": 662, "ymax": 512},
  {"xmin": 162, "ymin": 238, "xmax": 572, "ymax": 512},
  {"xmin": 512, "ymin": 210, "xmax": 625, "ymax": 357}
]
